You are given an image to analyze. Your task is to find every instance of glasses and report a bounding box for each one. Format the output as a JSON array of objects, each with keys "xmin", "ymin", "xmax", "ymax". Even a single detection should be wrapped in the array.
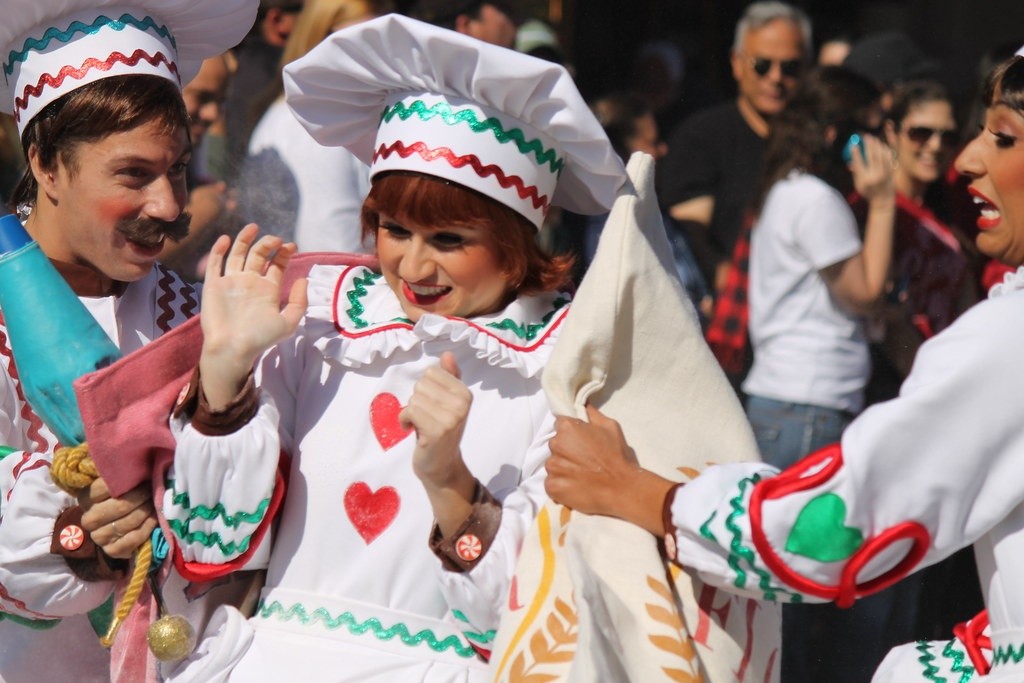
[
  {"xmin": 741, "ymin": 57, "xmax": 804, "ymax": 78},
  {"xmin": 899, "ymin": 125, "xmax": 961, "ymax": 148}
]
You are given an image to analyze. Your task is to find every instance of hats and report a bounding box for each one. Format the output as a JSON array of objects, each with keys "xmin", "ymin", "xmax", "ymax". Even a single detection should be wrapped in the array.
[
  {"xmin": 280, "ymin": 13, "xmax": 640, "ymax": 230},
  {"xmin": 0, "ymin": 0, "xmax": 260, "ymax": 141}
]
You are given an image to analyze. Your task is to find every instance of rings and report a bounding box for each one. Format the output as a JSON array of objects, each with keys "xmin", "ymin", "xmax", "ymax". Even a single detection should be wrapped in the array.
[{"xmin": 111, "ymin": 524, "xmax": 125, "ymax": 537}]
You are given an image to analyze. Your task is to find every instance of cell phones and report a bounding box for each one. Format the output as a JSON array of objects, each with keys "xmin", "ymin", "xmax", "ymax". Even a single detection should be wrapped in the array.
[{"xmin": 844, "ymin": 135, "xmax": 867, "ymax": 165}]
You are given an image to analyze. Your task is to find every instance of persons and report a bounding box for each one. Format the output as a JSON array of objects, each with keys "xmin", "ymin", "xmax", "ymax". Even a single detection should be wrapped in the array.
[
  {"xmin": 159, "ymin": 167, "xmax": 581, "ymax": 683},
  {"xmin": 438, "ymin": 0, "xmax": 1024, "ymax": 683},
  {"xmin": 0, "ymin": 0, "xmax": 390, "ymax": 683}
]
